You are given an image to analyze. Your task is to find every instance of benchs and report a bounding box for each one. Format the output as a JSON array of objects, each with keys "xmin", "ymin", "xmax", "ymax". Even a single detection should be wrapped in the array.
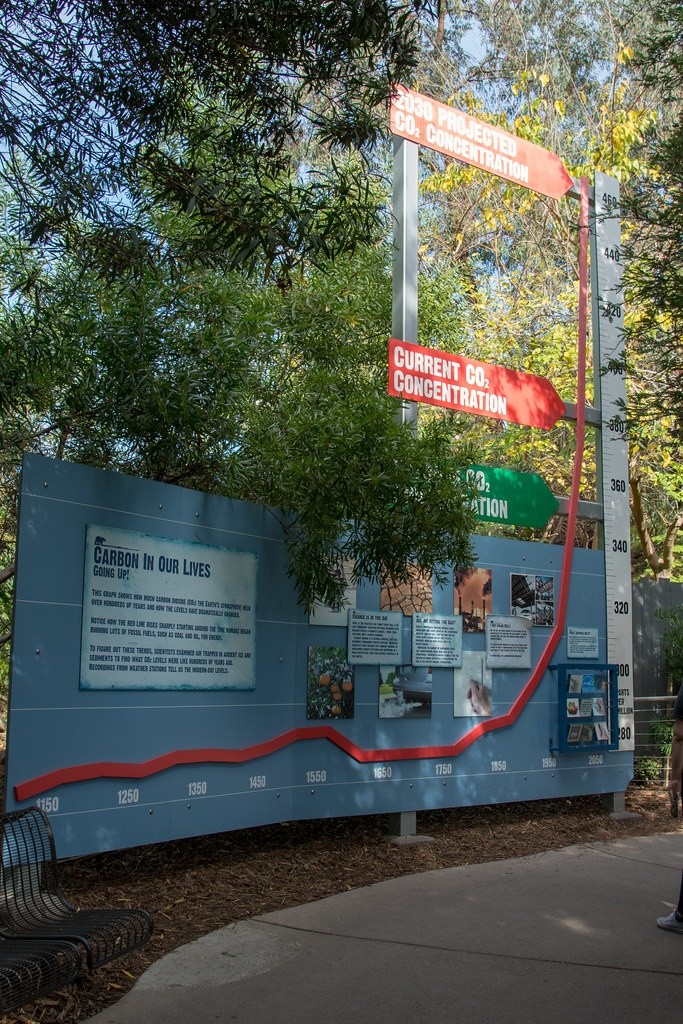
[{"xmin": 0, "ymin": 806, "xmax": 151, "ymax": 1014}]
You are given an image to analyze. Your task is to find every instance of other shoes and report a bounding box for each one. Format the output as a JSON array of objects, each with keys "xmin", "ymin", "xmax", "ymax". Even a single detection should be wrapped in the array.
[{"xmin": 656, "ymin": 912, "xmax": 683, "ymax": 934}]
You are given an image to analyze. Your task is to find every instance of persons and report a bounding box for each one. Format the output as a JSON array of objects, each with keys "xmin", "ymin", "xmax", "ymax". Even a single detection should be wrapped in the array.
[{"xmin": 656, "ymin": 682, "xmax": 683, "ymax": 933}]
